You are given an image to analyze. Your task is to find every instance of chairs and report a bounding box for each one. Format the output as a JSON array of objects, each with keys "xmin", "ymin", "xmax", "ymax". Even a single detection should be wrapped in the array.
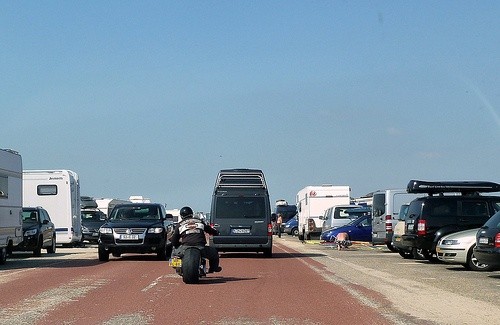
[
  {"xmin": 92, "ymin": 213, "xmax": 100, "ymax": 218},
  {"xmin": 29, "ymin": 212, "xmax": 37, "ymax": 222}
]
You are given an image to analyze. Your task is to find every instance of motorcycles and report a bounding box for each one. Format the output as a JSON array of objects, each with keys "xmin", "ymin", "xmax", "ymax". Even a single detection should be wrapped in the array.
[{"xmin": 164, "ymin": 222, "xmax": 220, "ymax": 283}]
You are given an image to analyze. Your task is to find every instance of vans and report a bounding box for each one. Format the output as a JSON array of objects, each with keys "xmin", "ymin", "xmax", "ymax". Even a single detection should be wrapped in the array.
[
  {"xmin": 209, "ymin": 168, "xmax": 274, "ymax": 256},
  {"xmin": 319, "ymin": 205, "xmax": 365, "ymax": 234},
  {"xmin": 284, "ymin": 213, "xmax": 299, "ymax": 235},
  {"xmin": 371, "ymin": 187, "xmax": 459, "ymax": 252},
  {"xmin": 275, "ymin": 199, "xmax": 298, "ymax": 233}
]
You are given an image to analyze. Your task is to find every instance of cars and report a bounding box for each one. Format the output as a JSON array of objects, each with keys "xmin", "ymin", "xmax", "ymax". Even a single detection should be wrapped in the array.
[
  {"xmin": 271, "ymin": 212, "xmax": 278, "ymax": 235},
  {"xmin": 472, "ymin": 210, "xmax": 500, "ymax": 264},
  {"xmin": 81, "ymin": 196, "xmax": 109, "ymax": 243},
  {"xmin": 319, "ymin": 215, "xmax": 372, "ymax": 243},
  {"xmin": 391, "ymin": 201, "xmax": 432, "ymax": 259},
  {"xmin": 435, "ymin": 227, "xmax": 494, "ymax": 271}
]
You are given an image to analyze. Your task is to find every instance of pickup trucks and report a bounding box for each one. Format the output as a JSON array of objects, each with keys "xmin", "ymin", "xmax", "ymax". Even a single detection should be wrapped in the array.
[{"xmin": 98, "ymin": 203, "xmax": 176, "ymax": 262}]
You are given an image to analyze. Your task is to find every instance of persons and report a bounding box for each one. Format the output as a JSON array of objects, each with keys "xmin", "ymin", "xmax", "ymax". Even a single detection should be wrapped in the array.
[
  {"xmin": 335, "ymin": 231, "xmax": 353, "ymax": 251},
  {"xmin": 277, "ymin": 213, "xmax": 282, "ymax": 238},
  {"xmin": 30, "ymin": 212, "xmax": 36, "ymax": 219},
  {"xmin": 170, "ymin": 207, "xmax": 223, "ymax": 273}
]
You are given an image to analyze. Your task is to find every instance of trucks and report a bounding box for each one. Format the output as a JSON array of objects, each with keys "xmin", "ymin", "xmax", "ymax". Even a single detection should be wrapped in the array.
[
  {"xmin": 22, "ymin": 169, "xmax": 82, "ymax": 244},
  {"xmin": 96, "ymin": 197, "xmax": 133, "ymax": 219},
  {"xmin": 295, "ymin": 184, "xmax": 351, "ymax": 240},
  {"xmin": 0, "ymin": 148, "xmax": 24, "ymax": 264}
]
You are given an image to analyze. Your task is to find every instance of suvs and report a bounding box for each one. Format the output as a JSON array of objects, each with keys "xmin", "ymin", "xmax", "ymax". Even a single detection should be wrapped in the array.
[
  {"xmin": 401, "ymin": 178, "xmax": 500, "ymax": 263},
  {"xmin": 6, "ymin": 206, "xmax": 57, "ymax": 256}
]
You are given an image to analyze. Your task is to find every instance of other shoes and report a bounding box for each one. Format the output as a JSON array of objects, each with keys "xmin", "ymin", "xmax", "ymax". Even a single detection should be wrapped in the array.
[
  {"xmin": 337, "ymin": 245, "xmax": 342, "ymax": 248},
  {"xmin": 345, "ymin": 246, "xmax": 348, "ymax": 248},
  {"xmin": 208, "ymin": 267, "xmax": 222, "ymax": 273},
  {"xmin": 176, "ymin": 268, "xmax": 181, "ymax": 272}
]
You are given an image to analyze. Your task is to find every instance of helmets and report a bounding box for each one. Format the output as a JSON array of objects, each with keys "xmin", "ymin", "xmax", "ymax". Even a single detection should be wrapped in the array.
[{"xmin": 180, "ymin": 207, "xmax": 193, "ymax": 219}]
